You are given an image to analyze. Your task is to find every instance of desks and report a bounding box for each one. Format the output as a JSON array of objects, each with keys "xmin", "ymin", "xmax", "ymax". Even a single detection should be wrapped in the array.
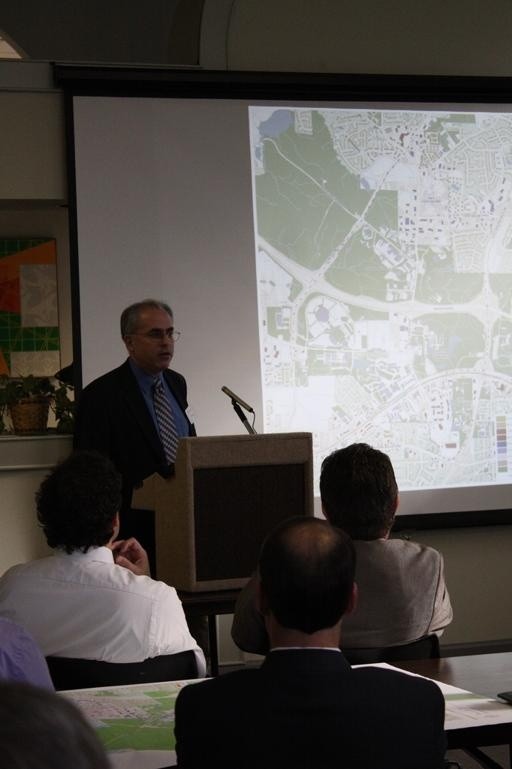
[
  {"xmin": 169, "ymin": 588, "xmax": 249, "ymax": 677},
  {"xmin": 55, "ymin": 661, "xmax": 512, "ymax": 769}
]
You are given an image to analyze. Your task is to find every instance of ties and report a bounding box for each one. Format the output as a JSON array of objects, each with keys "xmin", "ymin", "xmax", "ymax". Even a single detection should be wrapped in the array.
[{"xmin": 150, "ymin": 375, "xmax": 181, "ymax": 466}]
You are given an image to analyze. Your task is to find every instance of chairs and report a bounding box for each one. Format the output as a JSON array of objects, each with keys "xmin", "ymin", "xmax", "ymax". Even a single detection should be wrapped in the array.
[
  {"xmin": 336, "ymin": 634, "xmax": 440, "ymax": 663},
  {"xmin": 44, "ymin": 650, "xmax": 198, "ymax": 690}
]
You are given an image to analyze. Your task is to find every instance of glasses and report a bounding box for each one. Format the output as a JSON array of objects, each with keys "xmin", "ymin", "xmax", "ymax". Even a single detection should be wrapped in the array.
[{"xmin": 124, "ymin": 331, "xmax": 181, "ymax": 345}]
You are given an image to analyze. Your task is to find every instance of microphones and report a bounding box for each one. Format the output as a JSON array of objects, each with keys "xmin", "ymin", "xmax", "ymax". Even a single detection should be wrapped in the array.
[{"xmin": 220, "ymin": 385, "xmax": 253, "ymax": 413}]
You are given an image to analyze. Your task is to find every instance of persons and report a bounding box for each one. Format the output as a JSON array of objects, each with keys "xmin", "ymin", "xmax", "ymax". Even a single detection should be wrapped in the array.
[
  {"xmin": 231, "ymin": 444, "xmax": 456, "ymax": 656},
  {"xmin": 74, "ymin": 299, "xmax": 196, "ymax": 580},
  {"xmin": 171, "ymin": 517, "xmax": 445, "ymax": 768},
  {"xmin": 1, "ymin": 458, "xmax": 209, "ymax": 768}
]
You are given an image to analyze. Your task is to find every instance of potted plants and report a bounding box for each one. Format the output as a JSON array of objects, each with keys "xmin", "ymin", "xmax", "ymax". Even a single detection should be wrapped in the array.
[{"xmin": 0, "ymin": 372, "xmax": 80, "ymax": 437}]
